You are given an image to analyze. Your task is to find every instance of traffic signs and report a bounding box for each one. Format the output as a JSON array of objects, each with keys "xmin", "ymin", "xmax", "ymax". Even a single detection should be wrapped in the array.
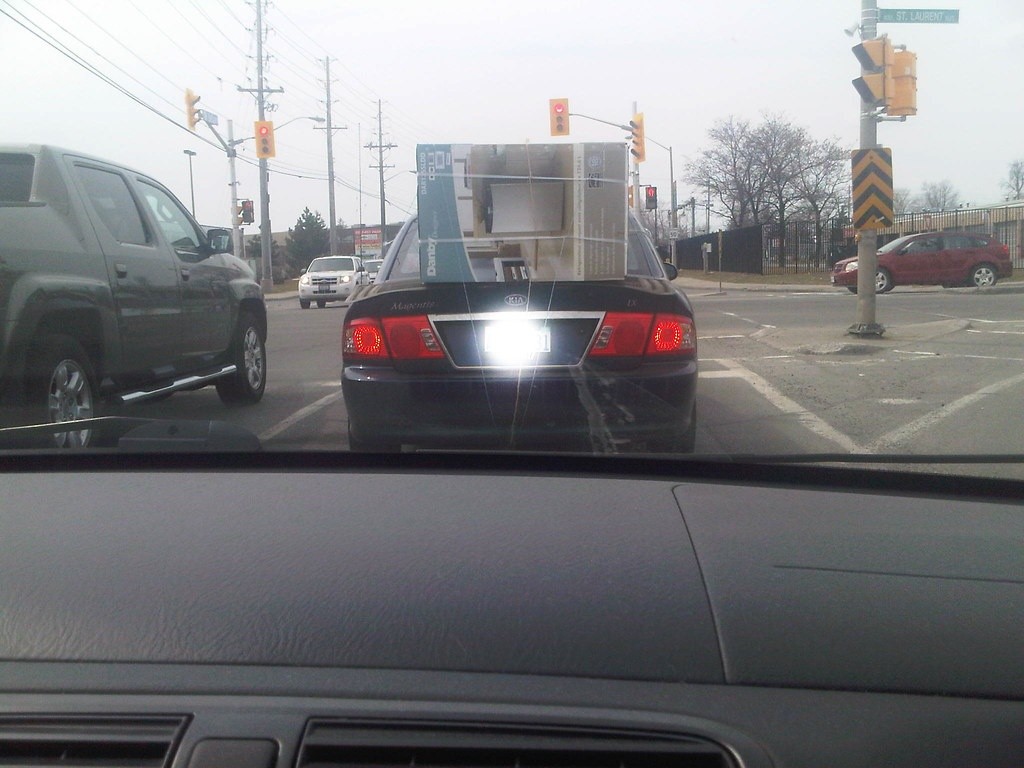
[{"xmin": 877, "ymin": 9, "xmax": 959, "ymax": 24}]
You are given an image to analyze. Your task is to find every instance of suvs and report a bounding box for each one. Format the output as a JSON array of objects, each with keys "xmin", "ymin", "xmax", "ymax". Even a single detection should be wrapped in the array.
[{"xmin": 297, "ymin": 254, "xmax": 369, "ymax": 308}]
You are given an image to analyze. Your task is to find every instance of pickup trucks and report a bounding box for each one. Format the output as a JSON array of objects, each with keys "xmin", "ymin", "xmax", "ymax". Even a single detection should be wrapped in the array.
[{"xmin": 0, "ymin": 144, "xmax": 268, "ymax": 450}]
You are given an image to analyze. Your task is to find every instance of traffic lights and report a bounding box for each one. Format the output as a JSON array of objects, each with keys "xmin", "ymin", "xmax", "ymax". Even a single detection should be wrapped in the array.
[
  {"xmin": 254, "ymin": 120, "xmax": 276, "ymax": 158},
  {"xmin": 236, "ymin": 206, "xmax": 243, "ymax": 227},
  {"xmin": 550, "ymin": 98, "xmax": 569, "ymax": 136},
  {"xmin": 629, "ymin": 112, "xmax": 645, "ymax": 163},
  {"xmin": 851, "ymin": 36, "xmax": 892, "ymax": 107},
  {"xmin": 646, "ymin": 186, "xmax": 656, "ymax": 210},
  {"xmin": 242, "ymin": 200, "xmax": 254, "ymax": 223},
  {"xmin": 187, "ymin": 90, "xmax": 201, "ymax": 130}
]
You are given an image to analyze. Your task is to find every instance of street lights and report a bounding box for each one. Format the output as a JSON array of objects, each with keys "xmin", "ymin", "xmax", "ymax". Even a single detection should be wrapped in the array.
[
  {"xmin": 229, "ymin": 116, "xmax": 325, "ymax": 252},
  {"xmin": 379, "ymin": 170, "xmax": 418, "ymax": 258},
  {"xmin": 183, "ymin": 149, "xmax": 196, "ymax": 218},
  {"xmin": 705, "ymin": 203, "xmax": 714, "ymax": 235}
]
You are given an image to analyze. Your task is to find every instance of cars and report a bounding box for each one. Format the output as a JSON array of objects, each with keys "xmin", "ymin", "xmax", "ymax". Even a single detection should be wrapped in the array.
[
  {"xmin": 830, "ymin": 229, "xmax": 1013, "ymax": 294},
  {"xmin": 341, "ymin": 211, "xmax": 700, "ymax": 454},
  {"xmin": 363, "ymin": 259, "xmax": 385, "ymax": 282}
]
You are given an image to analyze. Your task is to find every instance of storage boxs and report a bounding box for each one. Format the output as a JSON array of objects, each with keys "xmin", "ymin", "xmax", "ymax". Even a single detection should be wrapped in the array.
[{"xmin": 415, "ymin": 140, "xmax": 630, "ymax": 284}]
